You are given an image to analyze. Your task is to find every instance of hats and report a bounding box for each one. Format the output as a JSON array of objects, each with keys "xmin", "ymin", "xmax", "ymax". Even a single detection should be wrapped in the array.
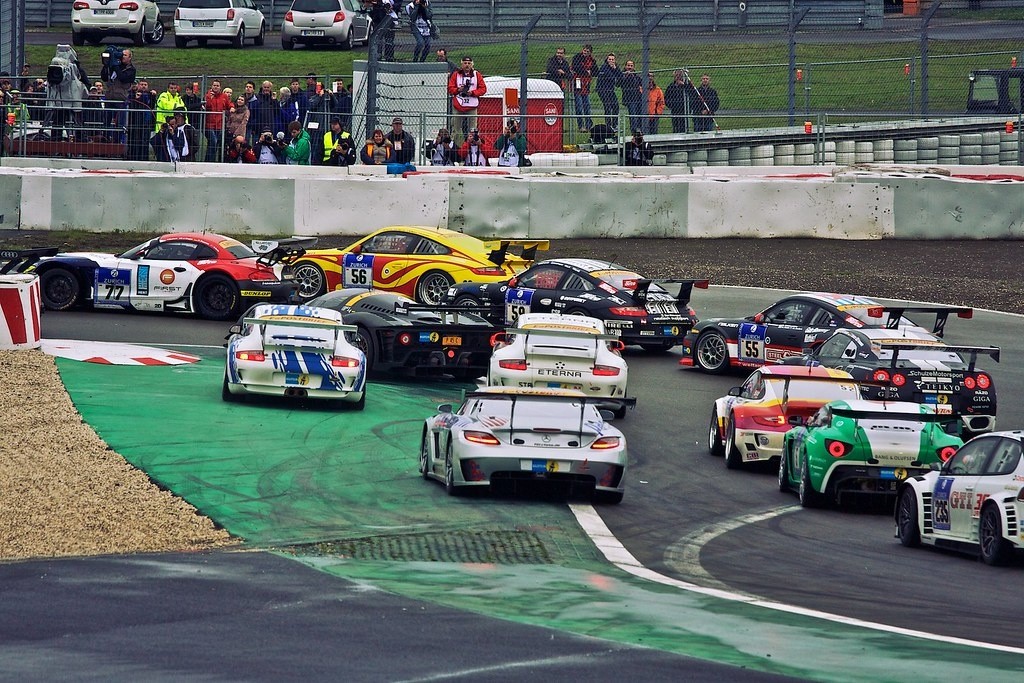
[
  {"xmin": 389, "ymin": 118, "xmax": 405, "ymax": 126},
  {"xmin": 89, "ymin": 86, "xmax": 98, "ymax": 91},
  {"xmin": 260, "ymin": 125, "xmax": 273, "ymax": 134},
  {"xmin": 462, "ymin": 56, "xmax": 472, "ymax": 61},
  {"xmin": 328, "ymin": 116, "xmax": 342, "ymax": 125},
  {"xmin": 173, "ymin": 106, "xmax": 186, "ymax": 117}
]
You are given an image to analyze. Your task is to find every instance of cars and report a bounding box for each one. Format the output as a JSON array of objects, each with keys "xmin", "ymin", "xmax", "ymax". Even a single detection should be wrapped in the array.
[
  {"xmin": 173, "ymin": 0, "xmax": 266, "ymax": 49},
  {"xmin": 71, "ymin": 0, "xmax": 166, "ymax": 47},
  {"xmin": 281, "ymin": 0, "xmax": 373, "ymax": 51}
]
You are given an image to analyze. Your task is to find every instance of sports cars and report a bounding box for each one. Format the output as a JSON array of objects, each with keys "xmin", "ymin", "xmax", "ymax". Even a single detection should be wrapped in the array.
[
  {"xmin": 300, "ymin": 288, "xmax": 508, "ymax": 382},
  {"xmin": 439, "ymin": 257, "xmax": 709, "ymax": 354},
  {"xmin": 19, "ymin": 231, "xmax": 319, "ymax": 320},
  {"xmin": 418, "ymin": 385, "xmax": 627, "ymax": 504},
  {"xmin": 707, "ymin": 365, "xmax": 864, "ymax": 471},
  {"xmin": 486, "ymin": 312, "xmax": 628, "ymax": 421},
  {"xmin": 892, "ymin": 430, "xmax": 1024, "ymax": 565},
  {"xmin": 801, "ymin": 328, "xmax": 1001, "ymax": 442},
  {"xmin": 677, "ymin": 293, "xmax": 974, "ymax": 374},
  {"xmin": 778, "ymin": 400, "xmax": 966, "ymax": 517},
  {"xmin": 222, "ymin": 303, "xmax": 368, "ymax": 410},
  {"xmin": 280, "ymin": 224, "xmax": 550, "ymax": 308}
]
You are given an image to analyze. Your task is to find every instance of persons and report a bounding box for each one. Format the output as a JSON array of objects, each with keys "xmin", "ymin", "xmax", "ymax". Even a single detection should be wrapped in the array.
[
  {"xmin": 361, "ymin": 0, "xmax": 433, "ymax": 62},
  {"xmin": 448, "ymin": 57, "xmax": 486, "ymax": 148},
  {"xmin": 620, "ymin": 131, "xmax": 654, "ymax": 165},
  {"xmin": 596, "ymin": 52, "xmax": 623, "ymax": 133},
  {"xmin": 664, "ymin": 70, "xmax": 718, "ymax": 133},
  {"xmin": 426, "ymin": 129, "xmax": 461, "ymax": 165},
  {"xmin": 547, "ymin": 47, "xmax": 573, "ymax": 133},
  {"xmin": 0, "ymin": 44, "xmax": 156, "ymax": 158},
  {"xmin": 621, "ymin": 60, "xmax": 642, "ymax": 134},
  {"xmin": 570, "ymin": 45, "xmax": 598, "ymax": 133},
  {"xmin": 493, "ymin": 119, "xmax": 527, "ymax": 166},
  {"xmin": 388, "ymin": 118, "xmax": 415, "ymax": 163},
  {"xmin": 149, "ymin": 73, "xmax": 356, "ymax": 166},
  {"xmin": 360, "ymin": 130, "xmax": 394, "ymax": 165},
  {"xmin": 457, "ymin": 128, "xmax": 488, "ymax": 166},
  {"xmin": 436, "ymin": 49, "xmax": 457, "ymax": 77},
  {"xmin": 640, "ymin": 73, "xmax": 664, "ymax": 134}
]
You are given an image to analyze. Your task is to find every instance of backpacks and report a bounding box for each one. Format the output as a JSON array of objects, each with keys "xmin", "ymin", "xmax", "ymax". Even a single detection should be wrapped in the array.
[{"xmin": 183, "ymin": 125, "xmax": 208, "ymax": 162}]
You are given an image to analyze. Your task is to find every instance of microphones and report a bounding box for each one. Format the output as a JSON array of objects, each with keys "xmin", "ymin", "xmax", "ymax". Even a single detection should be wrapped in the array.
[{"xmin": 277, "ymin": 132, "xmax": 285, "ymax": 139}]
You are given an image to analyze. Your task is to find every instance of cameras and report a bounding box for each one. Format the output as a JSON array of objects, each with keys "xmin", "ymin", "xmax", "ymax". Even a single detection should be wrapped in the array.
[
  {"xmin": 473, "ymin": 128, "xmax": 479, "ymax": 141},
  {"xmin": 162, "ymin": 123, "xmax": 168, "ymax": 128},
  {"xmin": 634, "ymin": 138, "xmax": 642, "ymax": 144},
  {"xmin": 439, "ymin": 136, "xmax": 451, "ymax": 145},
  {"xmin": 240, "ymin": 143, "xmax": 252, "ymax": 150},
  {"xmin": 264, "ymin": 135, "xmax": 271, "ymax": 142},
  {"xmin": 508, "ymin": 119, "xmax": 517, "ymax": 134},
  {"xmin": 462, "ymin": 85, "xmax": 470, "ymax": 97},
  {"xmin": 420, "ymin": 0, "xmax": 426, "ymax": 5},
  {"xmin": 384, "ymin": 3, "xmax": 391, "ymax": 9}
]
